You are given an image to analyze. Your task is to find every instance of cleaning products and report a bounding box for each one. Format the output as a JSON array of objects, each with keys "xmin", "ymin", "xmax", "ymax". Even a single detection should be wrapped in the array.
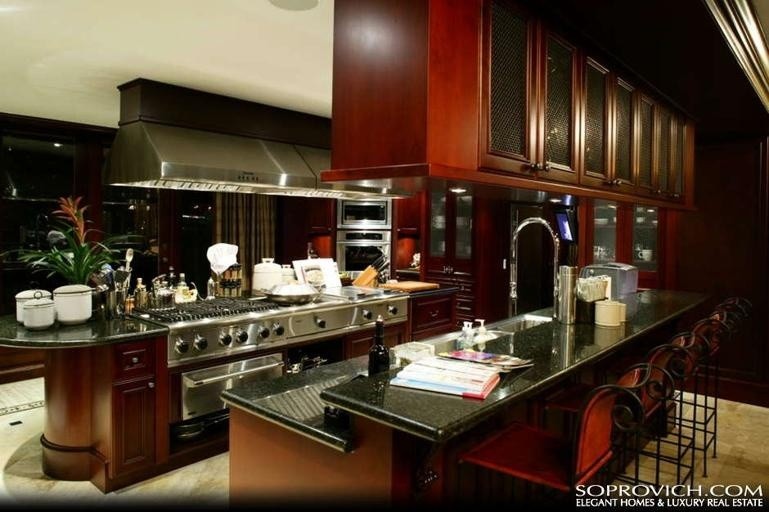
[{"xmin": 456, "ymin": 319, "xmax": 489, "ymax": 350}]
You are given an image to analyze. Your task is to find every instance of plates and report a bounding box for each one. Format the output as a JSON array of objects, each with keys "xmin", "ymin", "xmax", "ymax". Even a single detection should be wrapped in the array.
[
  {"xmin": 434, "ymin": 216, "xmax": 471, "ymax": 258},
  {"xmin": 266, "ymin": 286, "xmax": 321, "ymax": 303}
]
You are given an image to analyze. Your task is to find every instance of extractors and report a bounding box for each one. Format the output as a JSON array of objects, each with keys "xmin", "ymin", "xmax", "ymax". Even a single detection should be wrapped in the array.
[{"xmin": 103, "ymin": 76, "xmax": 416, "ymax": 202}]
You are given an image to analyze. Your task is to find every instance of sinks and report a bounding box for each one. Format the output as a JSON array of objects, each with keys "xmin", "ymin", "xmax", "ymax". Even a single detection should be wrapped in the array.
[
  {"xmin": 498, "ymin": 314, "xmax": 551, "ymax": 333},
  {"xmin": 408, "ymin": 335, "xmax": 499, "ymax": 356}
]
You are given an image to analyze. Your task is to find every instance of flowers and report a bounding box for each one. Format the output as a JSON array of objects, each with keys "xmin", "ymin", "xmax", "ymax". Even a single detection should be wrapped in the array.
[{"xmin": 0, "ymin": 196, "xmax": 145, "ymax": 284}]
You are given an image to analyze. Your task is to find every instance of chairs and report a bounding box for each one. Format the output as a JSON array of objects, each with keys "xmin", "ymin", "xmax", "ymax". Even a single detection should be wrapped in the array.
[{"xmin": 444, "ymin": 296, "xmax": 755, "ymax": 509}]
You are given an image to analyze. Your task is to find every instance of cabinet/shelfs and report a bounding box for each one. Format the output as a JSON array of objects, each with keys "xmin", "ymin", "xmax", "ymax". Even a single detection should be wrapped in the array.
[
  {"xmin": 420, "ymin": 189, "xmax": 507, "ymax": 330},
  {"xmin": 577, "ymin": 195, "xmax": 676, "ymax": 288},
  {"xmin": 89, "ymin": 335, "xmax": 168, "ymax": 492},
  {"xmin": 321, "ymin": 1, "xmax": 697, "ymax": 212}
]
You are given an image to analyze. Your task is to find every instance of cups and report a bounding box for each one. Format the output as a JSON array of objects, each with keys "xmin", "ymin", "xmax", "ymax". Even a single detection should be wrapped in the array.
[
  {"xmin": 638, "ymin": 250, "xmax": 653, "ymax": 261},
  {"xmin": 593, "ymin": 301, "xmax": 627, "ymax": 327}
]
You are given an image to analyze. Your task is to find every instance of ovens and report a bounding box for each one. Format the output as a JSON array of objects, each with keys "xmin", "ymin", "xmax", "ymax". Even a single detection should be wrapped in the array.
[{"xmin": 338, "ymin": 201, "xmax": 392, "ymax": 278}]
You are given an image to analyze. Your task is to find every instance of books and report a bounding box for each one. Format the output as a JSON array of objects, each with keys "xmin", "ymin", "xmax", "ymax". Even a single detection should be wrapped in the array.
[{"xmin": 389, "ymin": 350, "xmax": 534, "ymax": 400}]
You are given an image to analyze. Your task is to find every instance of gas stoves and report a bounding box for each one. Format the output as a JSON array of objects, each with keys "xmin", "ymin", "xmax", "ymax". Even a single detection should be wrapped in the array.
[{"xmin": 116, "ymin": 283, "xmax": 409, "ymax": 369}]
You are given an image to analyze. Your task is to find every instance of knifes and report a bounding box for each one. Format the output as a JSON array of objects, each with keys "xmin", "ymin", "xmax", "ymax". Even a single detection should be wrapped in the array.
[{"xmin": 373, "ymin": 255, "xmax": 389, "ymax": 272}]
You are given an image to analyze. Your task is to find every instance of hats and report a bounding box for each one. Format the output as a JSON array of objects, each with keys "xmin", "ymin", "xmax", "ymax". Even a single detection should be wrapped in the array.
[{"xmin": 207, "ymin": 243, "xmax": 239, "ymax": 276}]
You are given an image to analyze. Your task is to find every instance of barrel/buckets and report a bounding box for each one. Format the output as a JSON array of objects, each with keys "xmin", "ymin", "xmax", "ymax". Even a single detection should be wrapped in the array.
[{"xmin": 211, "ymin": 265, "xmax": 242, "ymax": 297}]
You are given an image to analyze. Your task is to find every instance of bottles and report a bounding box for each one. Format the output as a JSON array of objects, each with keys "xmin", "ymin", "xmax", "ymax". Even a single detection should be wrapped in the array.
[
  {"xmin": 558, "ymin": 266, "xmax": 576, "ymax": 324},
  {"xmin": 556, "ymin": 327, "xmax": 575, "ymax": 370},
  {"xmin": 368, "ymin": 318, "xmax": 388, "ymax": 375},
  {"xmin": 133, "ymin": 272, "xmax": 189, "ymax": 307},
  {"xmin": 206, "ymin": 263, "xmax": 242, "ymax": 299}
]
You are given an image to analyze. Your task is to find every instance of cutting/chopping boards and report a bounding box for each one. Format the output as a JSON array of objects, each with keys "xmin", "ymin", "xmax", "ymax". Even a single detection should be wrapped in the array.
[{"xmin": 379, "ymin": 281, "xmax": 439, "ymax": 291}]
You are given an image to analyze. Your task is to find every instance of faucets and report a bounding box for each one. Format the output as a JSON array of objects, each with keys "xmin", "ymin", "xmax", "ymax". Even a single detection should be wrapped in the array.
[{"xmin": 508, "ymin": 217, "xmax": 560, "ymax": 320}]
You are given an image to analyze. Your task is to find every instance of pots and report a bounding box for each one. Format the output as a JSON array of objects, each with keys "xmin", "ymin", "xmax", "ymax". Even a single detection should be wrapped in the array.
[{"xmin": 15, "ymin": 283, "xmax": 92, "ymax": 331}]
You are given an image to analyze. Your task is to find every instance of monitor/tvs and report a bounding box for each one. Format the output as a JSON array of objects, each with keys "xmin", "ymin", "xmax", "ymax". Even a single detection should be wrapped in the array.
[{"xmin": 554, "ymin": 208, "xmax": 578, "ymax": 245}]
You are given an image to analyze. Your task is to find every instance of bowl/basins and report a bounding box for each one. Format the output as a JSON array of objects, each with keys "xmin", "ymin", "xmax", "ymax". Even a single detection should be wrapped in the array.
[{"xmin": 595, "ymin": 218, "xmax": 609, "ymax": 225}]
